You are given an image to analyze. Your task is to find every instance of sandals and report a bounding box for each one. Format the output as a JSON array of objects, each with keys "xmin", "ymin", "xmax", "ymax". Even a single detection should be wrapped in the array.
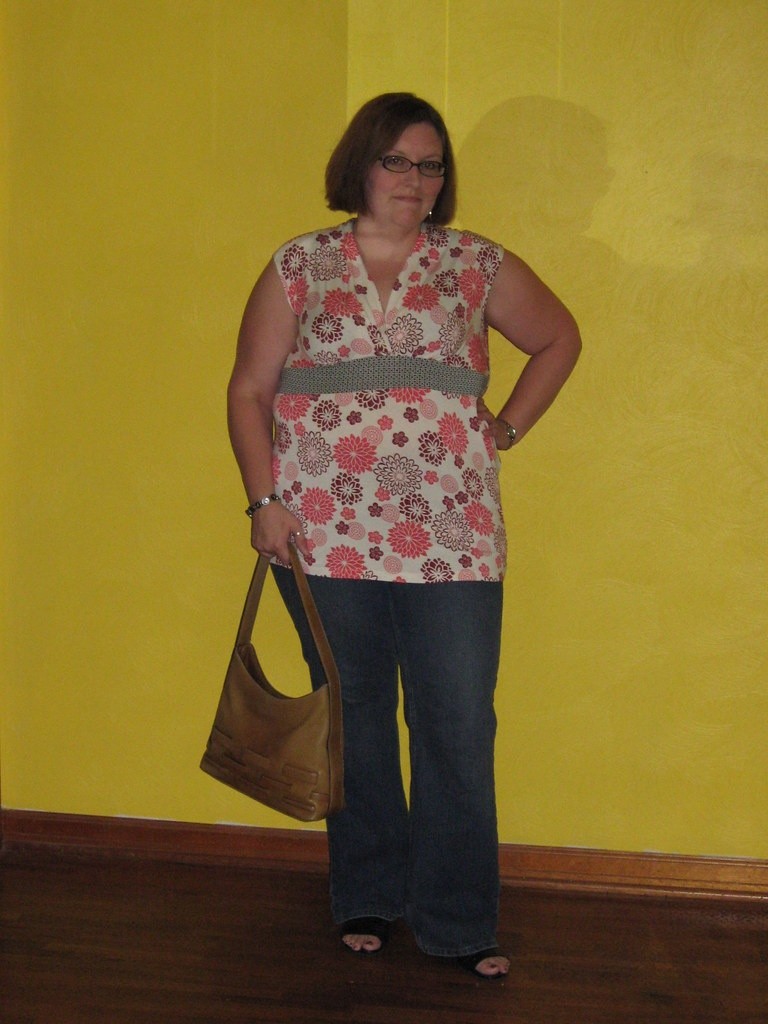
[
  {"xmin": 340, "ymin": 916, "xmax": 390, "ymax": 957},
  {"xmin": 458, "ymin": 947, "xmax": 511, "ymax": 981}
]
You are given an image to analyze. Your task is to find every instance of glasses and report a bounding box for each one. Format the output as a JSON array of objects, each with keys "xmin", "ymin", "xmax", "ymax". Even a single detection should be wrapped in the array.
[{"xmin": 377, "ymin": 155, "xmax": 448, "ymax": 177}]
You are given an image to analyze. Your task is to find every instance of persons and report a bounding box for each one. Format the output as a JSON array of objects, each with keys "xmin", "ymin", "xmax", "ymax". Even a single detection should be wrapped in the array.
[{"xmin": 225, "ymin": 90, "xmax": 583, "ymax": 983}]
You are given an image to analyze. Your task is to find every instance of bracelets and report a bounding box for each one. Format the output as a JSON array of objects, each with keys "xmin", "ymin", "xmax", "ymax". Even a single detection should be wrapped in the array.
[
  {"xmin": 496, "ymin": 418, "xmax": 517, "ymax": 452},
  {"xmin": 244, "ymin": 493, "xmax": 281, "ymax": 519}
]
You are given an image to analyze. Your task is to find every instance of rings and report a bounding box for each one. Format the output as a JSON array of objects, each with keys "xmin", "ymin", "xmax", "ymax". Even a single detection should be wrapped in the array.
[{"xmin": 292, "ymin": 528, "xmax": 308, "ymax": 536}]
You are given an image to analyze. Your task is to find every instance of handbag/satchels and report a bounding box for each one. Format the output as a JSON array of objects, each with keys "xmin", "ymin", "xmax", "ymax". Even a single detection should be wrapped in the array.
[{"xmin": 199, "ymin": 542, "xmax": 344, "ymax": 822}]
